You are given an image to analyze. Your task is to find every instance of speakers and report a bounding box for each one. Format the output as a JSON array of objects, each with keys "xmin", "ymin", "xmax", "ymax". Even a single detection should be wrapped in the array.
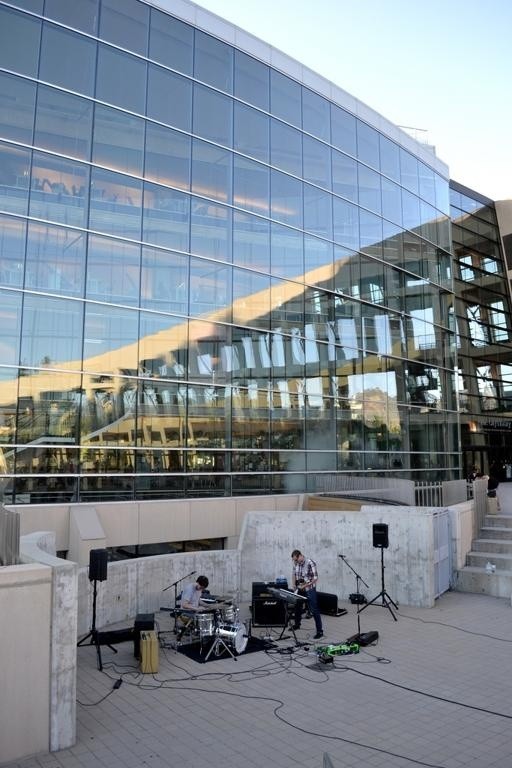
[
  {"xmin": 88, "ymin": 549, "xmax": 108, "ymax": 582},
  {"xmin": 373, "ymin": 523, "xmax": 388, "ymax": 548}
]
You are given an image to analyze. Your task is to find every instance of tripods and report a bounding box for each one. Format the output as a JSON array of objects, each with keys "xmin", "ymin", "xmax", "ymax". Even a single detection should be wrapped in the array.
[
  {"xmin": 205, "ymin": 623, "xmax": 237, "ymax": 663},
  {"xmin": 77, "ymin": 584, "xmax": 119, "ymax": 671},
  {"xmin": 357, "ymin": 550, "xmax": 404, "ymax": 623}
]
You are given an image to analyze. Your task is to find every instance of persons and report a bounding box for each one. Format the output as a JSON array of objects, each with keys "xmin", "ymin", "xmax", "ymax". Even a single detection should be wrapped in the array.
[
  {"xmin": 179, "ymin": 575, "xmax": 218, "ymax": 625},
  {"xmin": 469, "ymin": 461, "xmax": 484, "ymax": 480},
  {"xmin": 290, "ymin": 547, "xmax": 326, "ymax": 639}
]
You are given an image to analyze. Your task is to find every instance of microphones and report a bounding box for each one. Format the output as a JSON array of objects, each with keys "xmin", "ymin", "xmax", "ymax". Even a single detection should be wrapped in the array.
[
  {"xmin": 339, "ymin": 554, "xmax": 346, "ymax": 558},
  {"xmin": 187, "ymin": 570, "xmax": 197, "ymax": 577}
]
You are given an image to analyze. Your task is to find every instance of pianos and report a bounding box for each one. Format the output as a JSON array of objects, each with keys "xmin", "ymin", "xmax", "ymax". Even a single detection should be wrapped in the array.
[{"xmin": 269, "ymin": 587, "xmax": 307, "ymax": 605}]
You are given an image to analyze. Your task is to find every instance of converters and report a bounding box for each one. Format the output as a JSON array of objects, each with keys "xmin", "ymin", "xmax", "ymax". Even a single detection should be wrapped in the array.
[
  {"xmin": 319, "ymin": 654, "xmax": 333, "ymax": 664},
  {"xmin": 112, "ymin": 678, "xmax": 122, "ymax": 689}
]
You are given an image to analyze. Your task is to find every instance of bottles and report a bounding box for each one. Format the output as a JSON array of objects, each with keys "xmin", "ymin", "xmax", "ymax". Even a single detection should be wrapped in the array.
[{"xmin": 485, "ymin": 562, "xmax": 495, "ymax": 574}]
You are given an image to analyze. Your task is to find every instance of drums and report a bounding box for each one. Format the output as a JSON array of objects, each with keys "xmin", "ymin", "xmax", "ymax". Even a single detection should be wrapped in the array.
[
  {"xmin": 196, "ymin": 614, "xmax": 214, "ymax": 636},
  {"xmin": 218, "ymin": 604, "xmax": 238, "ymax": 623},
  {"xmin": 216, "ymin": 622, "xmax": 248, "ymax": 653}
]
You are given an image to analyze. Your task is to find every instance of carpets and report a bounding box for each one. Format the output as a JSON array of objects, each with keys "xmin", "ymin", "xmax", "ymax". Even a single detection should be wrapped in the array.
[{"xmin": 174, "ymin": 634, "xmax": 278, "ymax": 664}]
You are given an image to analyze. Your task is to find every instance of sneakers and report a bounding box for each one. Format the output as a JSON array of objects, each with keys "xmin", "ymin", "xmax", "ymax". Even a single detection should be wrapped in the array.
[
  {"xmin": 313, "ymin": 630, "xmax": 323, "ymax": 639},
  {"xmin": 288, "ymin": 624, "xmax": 300, "ymax": 632}
]
